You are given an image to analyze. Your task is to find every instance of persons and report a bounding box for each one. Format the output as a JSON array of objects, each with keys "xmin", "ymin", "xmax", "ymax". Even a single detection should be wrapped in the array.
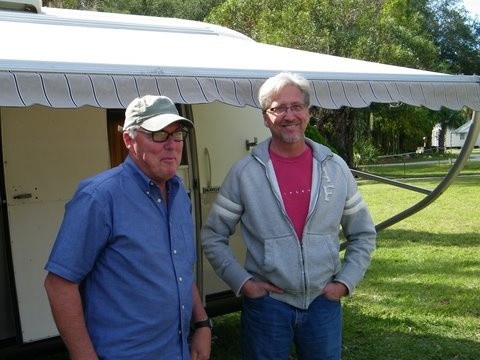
[
  {"xmin": 44, "ymin": 95, "xmax": 214, "ymax": 360},
  {"xmin": 353, "ymin": 152, "xmax": 360, "ymax": 168},
  {"xmin": 200, "ymin": 72, "xmax": 377, "ymax": 360}
]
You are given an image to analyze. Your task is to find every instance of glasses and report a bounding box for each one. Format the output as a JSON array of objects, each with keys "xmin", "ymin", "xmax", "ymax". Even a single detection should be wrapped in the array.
[
  {"xmin": 134, "ymin": 128, "xmax": 189, "ymax": 143},
  {"xmin": 266, "ymin": 103, "xmax": 308, "ymax": 117}
]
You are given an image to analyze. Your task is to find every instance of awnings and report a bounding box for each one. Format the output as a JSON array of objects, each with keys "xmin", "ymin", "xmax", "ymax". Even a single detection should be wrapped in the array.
[{"xmin": 0, "ymin": 7, "xmax": 480, "ymax": 110}]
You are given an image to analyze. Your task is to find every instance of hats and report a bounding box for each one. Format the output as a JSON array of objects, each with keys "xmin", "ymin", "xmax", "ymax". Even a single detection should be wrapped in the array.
[{"xmin": 123, "ymin": 95, "xmax": 195, "ymax": 134}]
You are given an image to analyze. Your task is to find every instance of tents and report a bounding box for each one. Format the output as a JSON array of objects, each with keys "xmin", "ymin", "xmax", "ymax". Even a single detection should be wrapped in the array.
[{"xmin": 449, "ymin": 119, "xmax": 480, "ymax": 164}]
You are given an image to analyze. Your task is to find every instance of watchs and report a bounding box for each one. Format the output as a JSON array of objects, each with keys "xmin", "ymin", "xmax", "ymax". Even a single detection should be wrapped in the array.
[{"xmin": 191, "ymin": 318, "xmax": 213, "ymax": 330}]
